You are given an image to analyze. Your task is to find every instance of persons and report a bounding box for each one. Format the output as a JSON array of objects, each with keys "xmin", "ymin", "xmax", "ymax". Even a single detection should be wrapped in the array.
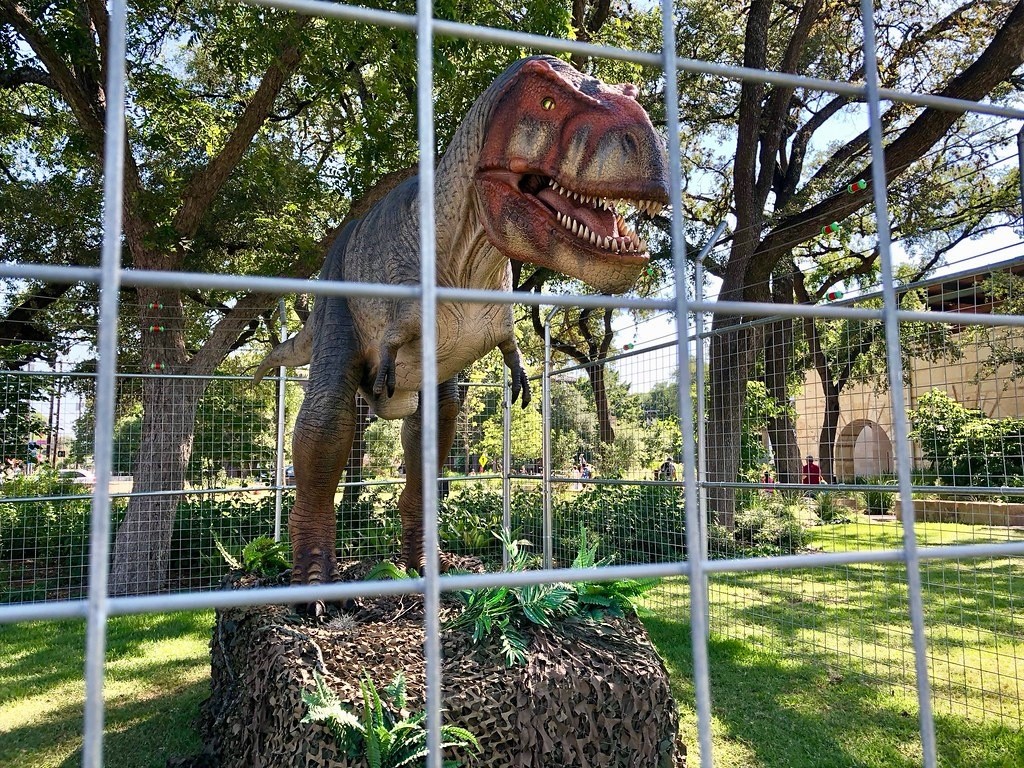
[
  {"xmin": 470, "ymin": 462, "xmax": 596, "ymax": 492},
  {"xmin": 660, "ymin": 457, "xmax": 678, "ymax": 481},
  {"xmin": 761, "ymin": 471, "xmax": 774, "ymax": 493},
  {"xmin": 802, "ymin": 456, "xmax": 821, "ymax": 500}
]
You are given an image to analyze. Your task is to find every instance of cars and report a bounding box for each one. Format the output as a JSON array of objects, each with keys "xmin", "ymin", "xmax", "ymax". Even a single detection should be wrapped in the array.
[
  {"xmin": 283, "ymin": 465, "xmax": 297, "ymax": 486},
  {"xmin": 55, "ymin": 469, "xmax": 95, "ymax": 484}
]
